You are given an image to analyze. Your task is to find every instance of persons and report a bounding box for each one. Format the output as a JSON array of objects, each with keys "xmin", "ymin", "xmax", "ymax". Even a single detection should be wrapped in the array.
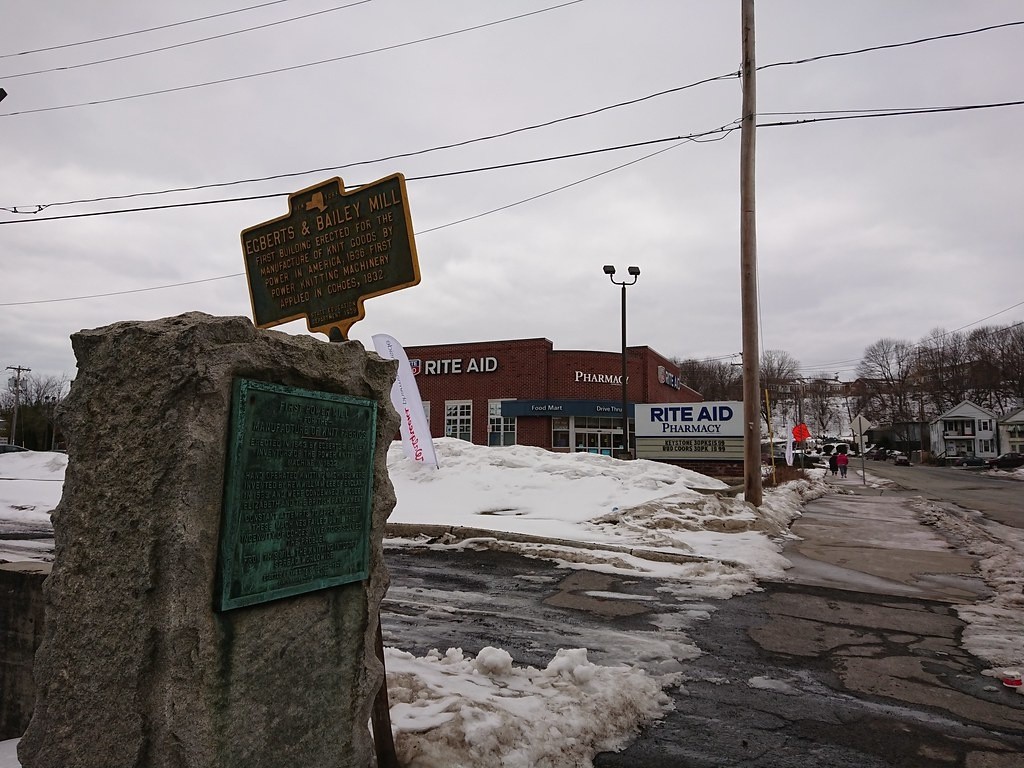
[
  {"xmin": 829, "ymin": 454, "xmax": 839, "ymax": 476},
  {"xmin": 837, "ymin": 452, "xmax": 849, "ymax": 478}
]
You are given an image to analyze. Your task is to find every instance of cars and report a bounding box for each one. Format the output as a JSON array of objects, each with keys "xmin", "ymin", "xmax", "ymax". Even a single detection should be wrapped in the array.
[
  {"xmin": 894, "ymin": 455, "xmax": 910, "ymax": 466},
  {"xmin": 954, "ymin": 456, "xmax": 990, "ymax": 467},
  {"xmin": 865, "ymin": 450, "xmax": 886, "ymax": 461},
  {"xmin": 990, "ymin": 451, "xmax": 1024, "ymax": 470}
]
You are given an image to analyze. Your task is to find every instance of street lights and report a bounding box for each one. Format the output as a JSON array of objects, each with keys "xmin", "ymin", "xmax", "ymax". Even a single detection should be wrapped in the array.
[{"xmin": 603, "ymin": 265, "xmax": 641, "ymax": 461}]
